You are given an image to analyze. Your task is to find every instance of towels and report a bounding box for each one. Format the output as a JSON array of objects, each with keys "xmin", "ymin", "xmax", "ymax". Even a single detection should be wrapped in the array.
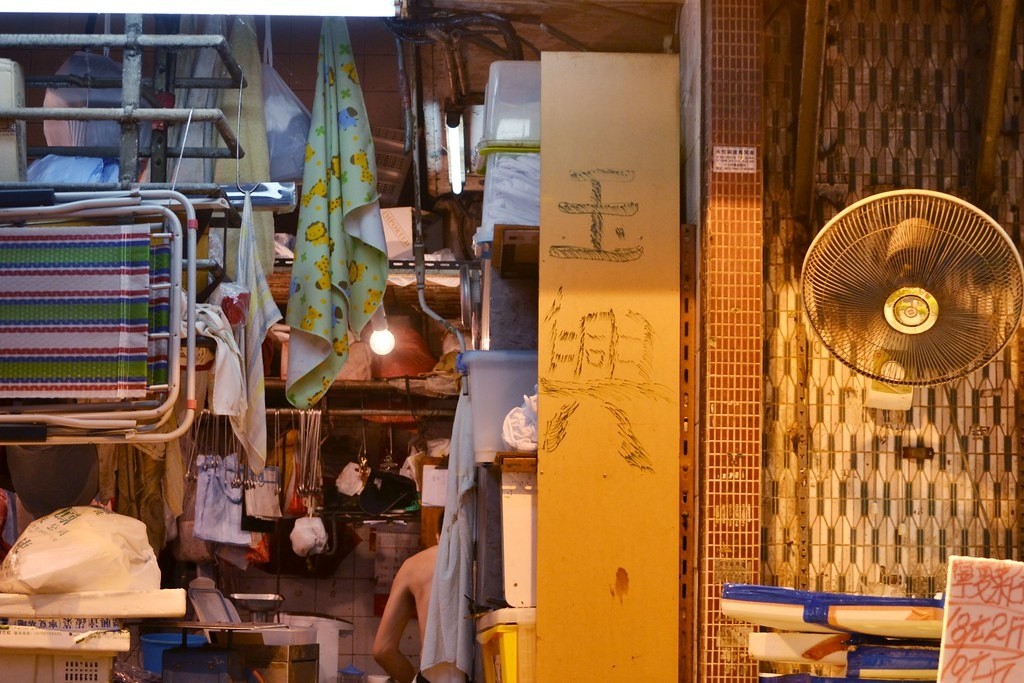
[
  {"xmin": 228, "ymin": 192, "xmax": 284, "ymax": 475},
  {"xmin": 420, "ymin": 382, "xmax": 475, "ymax": 683}
]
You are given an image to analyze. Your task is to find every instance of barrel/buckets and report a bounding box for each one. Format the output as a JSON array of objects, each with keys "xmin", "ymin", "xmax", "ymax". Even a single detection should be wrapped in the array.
[{"xmin": 137, "ymin": 635, "xmax": 207, "ymax": 675}]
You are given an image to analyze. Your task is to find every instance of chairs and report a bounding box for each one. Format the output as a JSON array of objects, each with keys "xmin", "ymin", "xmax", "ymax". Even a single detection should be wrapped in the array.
[{"xmin": 1, "ymin": 186, "xmax": 199, "ymax": 444}]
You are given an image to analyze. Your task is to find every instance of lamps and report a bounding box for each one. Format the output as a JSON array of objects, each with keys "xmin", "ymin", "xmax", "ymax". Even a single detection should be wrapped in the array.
[{"xmin": 443, "ymin": 99, "xmax": 466, "ymax": 195}]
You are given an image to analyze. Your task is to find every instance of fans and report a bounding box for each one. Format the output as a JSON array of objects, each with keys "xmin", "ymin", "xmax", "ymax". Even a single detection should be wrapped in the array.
[{"xmin": 798, "ymin": 188, "xmax": 1023, "ymax": 409}]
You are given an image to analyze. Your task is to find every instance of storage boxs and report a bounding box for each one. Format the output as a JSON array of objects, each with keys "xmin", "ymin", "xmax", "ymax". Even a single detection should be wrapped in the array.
[
  {"xmin": 455, "ymin": 349, "xmax": 538, "ymax": 464},
  {"xmin": 0, "ymin": 648, "xmax": 122, "ymax": 683},
  {"xmin": 469, "ymin": 458, "xmax": 535, "ymax": 683},
  {"xmin": 371, "ymin": 126, "xmax": 413, "ymax": 205},
  {"xmin": 472, "ymin": 61, "xmax": 540, "ymax": 256},
  {"xmin": 281, "ymin": 342, "xmax": 373, "ymax": 380},
  {"xmin": 208, "ymin": 626, "xmax": 322, "ymax": 683},
  {"xmin": 380, "ymin": 206, "xmax": 443, "ymax": 261}
]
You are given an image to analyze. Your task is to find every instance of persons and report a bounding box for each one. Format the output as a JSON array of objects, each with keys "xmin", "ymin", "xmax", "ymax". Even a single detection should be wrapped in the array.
[{"xmin": 373, "ymin": 512, "xmax": 467, "ymax": 683}]
